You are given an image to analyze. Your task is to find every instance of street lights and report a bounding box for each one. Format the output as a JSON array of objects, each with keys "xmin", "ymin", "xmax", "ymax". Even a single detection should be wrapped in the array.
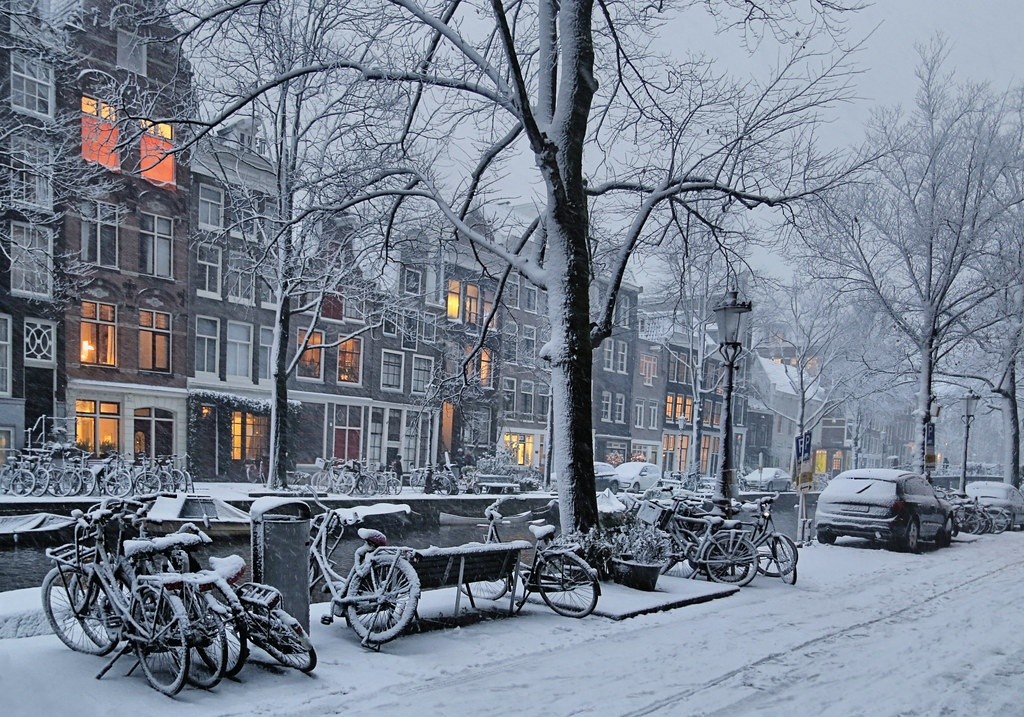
[
  {"xmin": 715, "ymin": 292, "xmax": 752, "ymax": 501},
  {"xmin": 678, "ymin": 413, "xmax": 688, "ymax": 480},
  {"xmin": 959, "ymin": 387, "xmax": 979, "ymax": 490}
]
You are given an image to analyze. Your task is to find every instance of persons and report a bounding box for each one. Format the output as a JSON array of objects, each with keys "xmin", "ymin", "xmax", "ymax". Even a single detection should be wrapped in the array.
[
  {"xmin": 392, "ymin": 455, "xmax": 403, "ymax": 490},
  {"xmin": 943, "ymin": 458, "xmax": 949, "ymax": 469},
  {"xmin": 893, "ymin": 458, "xmax": 898, "ymax": 470}
]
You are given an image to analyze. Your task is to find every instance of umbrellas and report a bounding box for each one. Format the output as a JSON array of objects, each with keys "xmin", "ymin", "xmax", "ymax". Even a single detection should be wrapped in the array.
[{"xmin": 887, "ymin": 455, "xmax": 898, "ymax": 463}]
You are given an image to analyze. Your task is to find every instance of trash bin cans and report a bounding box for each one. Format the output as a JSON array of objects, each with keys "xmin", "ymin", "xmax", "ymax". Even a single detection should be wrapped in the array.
[{"xmin": 249, "ymin": 494, "xmax": 312, "ymax": 642}]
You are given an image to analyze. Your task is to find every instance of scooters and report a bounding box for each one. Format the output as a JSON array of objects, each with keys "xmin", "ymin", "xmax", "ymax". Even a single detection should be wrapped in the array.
[{"xmin": 738, "ymin": 477, "xmax": 751, "ymax": 491}]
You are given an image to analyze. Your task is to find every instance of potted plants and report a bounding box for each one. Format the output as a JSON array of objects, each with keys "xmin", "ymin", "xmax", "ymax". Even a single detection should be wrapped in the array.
[
  {"xmin": 611, "ymin": 524, "xmax": 670, "ymax": 592},
  {"xmin": 554, "ymin": 525, "xmax": 617, "ymax": 585}
]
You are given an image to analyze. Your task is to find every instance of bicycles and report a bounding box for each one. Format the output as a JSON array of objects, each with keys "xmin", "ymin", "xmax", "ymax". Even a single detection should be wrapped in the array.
[
  {"xmin": 459, "ymin": 496, "xmax": 602, "ymax": 618},
  {"xmin": 311, "ymin": 458, "xmax": 402, "ymax": 495},
  {"xmin": 410, "ymin": 469, "xmax": 452, "ymax": 496},
  {"xmin": 0, "ymin": 453, "xmax": 196, "ymax": 498},
  {"xmin": 937, "ymin": 490, "xmax": 1008, "ymax": 534},
  {"xmin": 632, "ymin": 497, "xmax": 799, "ymax": 585},
  {"xmin": 457, "ymin": 470, "xmax": 481, "ymax": 494},
  {"xmin": 41, "ymin": 499, "xmax": 317, "ymax": 696},
  {"xmin": 673, "ymin": 473, "xmax": 696, "ymax": 493},
  {"xmin": 247, "ymin": 459, "xmax": 266, "ymax": 484},
  {"xmin": 305, "ymin": 483, "xmax": 423, "ymax": 653}
]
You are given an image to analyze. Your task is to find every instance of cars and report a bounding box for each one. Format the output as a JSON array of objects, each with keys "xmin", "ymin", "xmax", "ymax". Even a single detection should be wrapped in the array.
[
  {"xmin": 550, "ymin": 462, "xmax": 620, "ymax": 494},
  {"xmin": 744, "ymin": 468, "xmax": 792, "ymax": 491},
  {"xmin": 815, "ymin": 469, "xmax": 953, "ymax": 549},
  {"xmin": 614, "ymin": 462, "xmax": 661, "ymax": 492},
  {"xmin": 956, "ymin": 482, "xmax": 1024, "ymax": 531}
]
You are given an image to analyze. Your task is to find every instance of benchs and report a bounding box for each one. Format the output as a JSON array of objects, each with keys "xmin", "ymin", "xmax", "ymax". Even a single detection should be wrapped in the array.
[
  {"xmin": 476, "ymin": 474, "xmax": 520, "ymax": 494},
  {"xmin": 384, "ymin": 541, "xmax": 534, "ymax": 623}
]
